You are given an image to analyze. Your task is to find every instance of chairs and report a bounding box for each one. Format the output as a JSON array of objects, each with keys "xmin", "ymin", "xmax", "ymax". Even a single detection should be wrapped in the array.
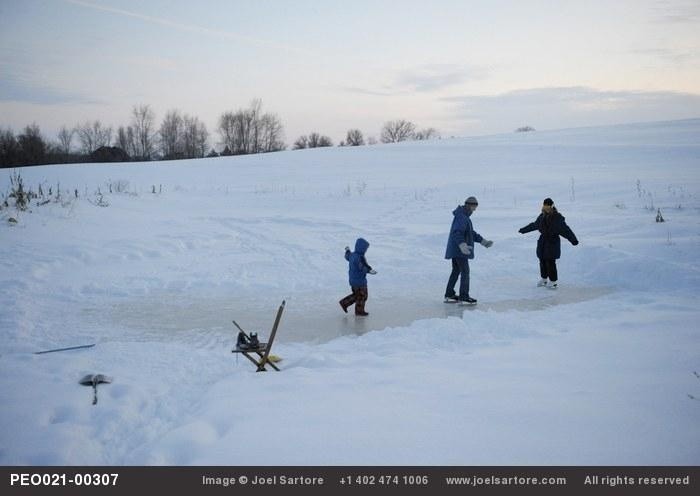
[{"xmin": 231, "ymin": 302, "xmax": 287, "ymax": 373}]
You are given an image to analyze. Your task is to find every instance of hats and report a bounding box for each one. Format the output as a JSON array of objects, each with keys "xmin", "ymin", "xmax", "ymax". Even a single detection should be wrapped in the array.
[{"xmin": 465, "ymin": 197, "xmax": 478, "ymax": 205}]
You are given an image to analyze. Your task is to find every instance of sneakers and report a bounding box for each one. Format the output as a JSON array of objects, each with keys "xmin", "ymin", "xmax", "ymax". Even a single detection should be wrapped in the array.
[
  {"xmin": 536, "ymin": 277, "xmax": 548, "ymax": 287},
  {"xmin": 457, "ymin": 296, "xmax": 477, "ymax": 305},
  {"xmin": 546, "ymin": 280, "xmax": 559, "ymax": 288},
  {"xmin": 445, "ymin": 294, "xmax": 459, "ymax": 303}
]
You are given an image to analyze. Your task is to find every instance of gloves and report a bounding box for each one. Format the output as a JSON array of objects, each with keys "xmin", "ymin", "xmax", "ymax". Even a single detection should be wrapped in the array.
[
  {"xmin": 459, "ymin": 243, "xmax": 473, "ymax": 255},
  {"xmin": 480, "ymin": 239, "xmax": 493, "ymax": 248}
]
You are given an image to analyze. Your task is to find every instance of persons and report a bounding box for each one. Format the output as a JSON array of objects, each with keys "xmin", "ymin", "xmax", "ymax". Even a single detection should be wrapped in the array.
[
  {"xmin": 519, "ymin": 197, "xmax": 581, "ymax": 289},
  {"xmin": 444, "ymin": 195, "xmax": 495, "ymax": 306},
  {"xmin": 339, "ymin": 237, "xmax": 376, "ymax": 314}
]
